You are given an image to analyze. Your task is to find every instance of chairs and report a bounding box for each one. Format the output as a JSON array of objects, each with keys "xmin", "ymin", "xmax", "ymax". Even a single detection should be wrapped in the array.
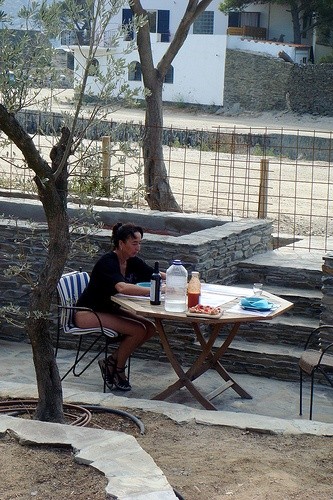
[
  {"xmin": 298, "ymin": 325, "xmax": 333, "ymax": 420},
  {"xmin": 55, "ymin": 267, "xmax": 131, "ymax": 394}
]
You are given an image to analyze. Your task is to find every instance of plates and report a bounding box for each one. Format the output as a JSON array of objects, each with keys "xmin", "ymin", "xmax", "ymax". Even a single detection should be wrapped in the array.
[{"xmin": 187, "ymin": 308, "xmax": 224, "ymax": 318}]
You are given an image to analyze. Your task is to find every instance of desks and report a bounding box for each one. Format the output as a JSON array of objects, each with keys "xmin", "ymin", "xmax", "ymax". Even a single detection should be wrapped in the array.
[{"xmin": 111, "ymin": 280, "xmax": 294, "ymax": 411}]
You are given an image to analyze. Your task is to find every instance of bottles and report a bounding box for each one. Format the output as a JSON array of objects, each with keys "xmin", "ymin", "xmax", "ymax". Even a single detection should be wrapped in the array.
[
  {"xmin": 150, "ymin": 262, "xmax": 163, "ymax": 304},
  {"xmin": 189, "ymin": 271, "xmax": 201, "ymax": 307},
  {"xmin": 164, "ymin": 260, "xmax": 188, "ymax": 312}
]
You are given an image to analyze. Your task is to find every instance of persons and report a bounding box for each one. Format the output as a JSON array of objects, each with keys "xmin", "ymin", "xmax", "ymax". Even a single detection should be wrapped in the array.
[{"xmin": 72, "ymin": 222, "xmax": 175, "ymax": 391}]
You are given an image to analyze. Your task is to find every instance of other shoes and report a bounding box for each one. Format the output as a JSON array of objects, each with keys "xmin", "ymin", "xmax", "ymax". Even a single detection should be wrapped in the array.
[
  {"xmin": 106, "ymin": 355, "xmax": 132, "ymax": 391},
  {"xmin": 98, "ymin": 359, "xmax": 113, "ymax": 389}
]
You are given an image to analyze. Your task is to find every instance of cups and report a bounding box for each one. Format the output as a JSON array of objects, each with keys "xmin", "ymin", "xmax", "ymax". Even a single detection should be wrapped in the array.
[{"xmin": 253, "ymin": 283, "xmax": 264, "ymax": 297}]
